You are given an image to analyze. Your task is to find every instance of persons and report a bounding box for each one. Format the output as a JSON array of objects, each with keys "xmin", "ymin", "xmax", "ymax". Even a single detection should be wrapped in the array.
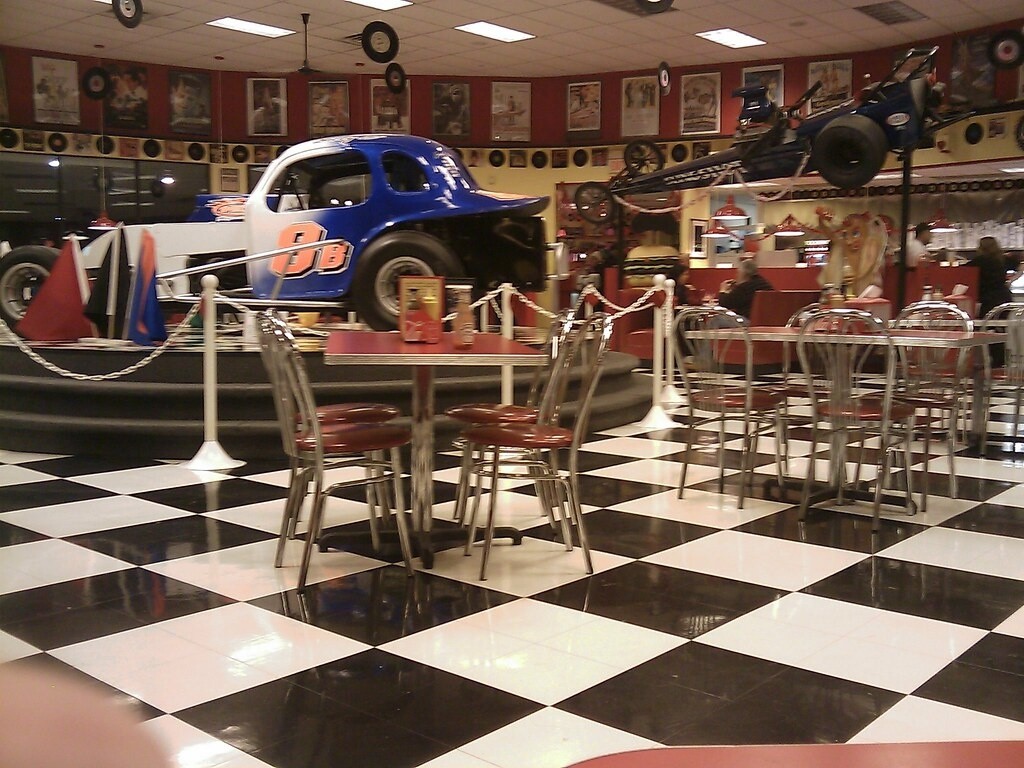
[
  {"xmin": 169, "ymin": 74, "xmax": 207, "ymax": 120},
  {"xmin": 507, "ymin": 95, "xmax": 516, "ymax": 123},
  {"xmin": 625, "ymin": 80, "xmax": 657, "ymax": 108},
  {"xmin": 43, "ymin": 235, "xmax": 57, "ymax": 247},
  {"xmin": 108, "ymin": 69, "xmax": 147, "ymax": 117},
  {"xmin": 574, "ymin": 91, "xmax": 584, "ymax": 108},
  {"xmin": 468, "ymin": 151, "xmax": 480, "ymax": 167},
  {"xmin": 905, "ymin": 222, "xmax": 933, "ymax": 268},
  {"xmin": 667, "ymin": 265, "xmax": 705, "ymax": 316},
  {"xmin": 960, "ymin": 236, "xmax": 1024, "ymax": 369},
  {"xmin": 719, "ymin": 258, "xmax": 775, "ymax": 318},
  {"xmin": 584, "ymin": 245, "xmax": 626, "ymax": 310}
]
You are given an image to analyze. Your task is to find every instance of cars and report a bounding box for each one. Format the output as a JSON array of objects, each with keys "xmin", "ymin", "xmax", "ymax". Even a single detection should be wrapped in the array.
[{"xmin": 1, "ymin": 134, "xmax": 551, "ymax": 340}]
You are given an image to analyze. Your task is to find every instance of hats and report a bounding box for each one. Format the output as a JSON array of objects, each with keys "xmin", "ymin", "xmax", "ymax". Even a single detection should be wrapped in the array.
[{"xmin": 916, "ymin": 222, "xmax": 933, "ymax": 232}]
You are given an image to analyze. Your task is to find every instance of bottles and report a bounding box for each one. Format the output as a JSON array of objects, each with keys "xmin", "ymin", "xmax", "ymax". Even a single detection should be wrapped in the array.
[
  {"xmin": 455, "ymin": 289, "xmax": 475, "ymax": 350},
  {"xmin": 922, "ymin": 285, "xmax": 935, "ymax": 300},
  {"xmin": 423, "ymin": 293, "xmax": 440, "ymax": 344},
  {"xmin": 403, "ymin": 287, "xmax": 423, "ymax": 345},
  {"xmin": 819, "ymin": 283, "xmax": 845, "ymax": 333}
]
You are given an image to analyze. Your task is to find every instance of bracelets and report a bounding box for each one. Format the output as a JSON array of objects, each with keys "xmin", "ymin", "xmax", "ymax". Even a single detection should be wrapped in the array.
[{"xmin": 1005, "ymin": 279, "xmax": 1012, "ymax": 283}]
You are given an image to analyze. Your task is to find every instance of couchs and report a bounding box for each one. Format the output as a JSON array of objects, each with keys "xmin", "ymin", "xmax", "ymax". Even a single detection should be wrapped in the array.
[
  {"xmin": 712, "ymin": 289, "xmax": 821, "ymax": 364},
  {"xmin": 617, "ymin": 288, "xmax": 669, "ymax": 361}
]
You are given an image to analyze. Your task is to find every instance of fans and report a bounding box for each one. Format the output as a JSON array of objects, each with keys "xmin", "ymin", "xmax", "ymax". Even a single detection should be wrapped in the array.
[{"xmin": 267, "ymin": 13, "xmax": 347, "ymax": 81}]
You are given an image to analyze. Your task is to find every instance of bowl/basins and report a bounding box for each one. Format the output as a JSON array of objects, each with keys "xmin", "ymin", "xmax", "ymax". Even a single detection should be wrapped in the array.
[{"xmin": 297, "ymin": 312, "xmax": 320, "ymax": 328}]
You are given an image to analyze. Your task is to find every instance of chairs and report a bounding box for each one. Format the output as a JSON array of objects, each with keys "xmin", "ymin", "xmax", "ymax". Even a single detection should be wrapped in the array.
[{"xmin": 254, "ymin": 300, "xmax": 1024, "ymax": 593}]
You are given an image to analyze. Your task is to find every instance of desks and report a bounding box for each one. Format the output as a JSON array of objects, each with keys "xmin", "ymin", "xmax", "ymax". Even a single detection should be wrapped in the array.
[
  {"xmin": 686, "ymin": 326, "xmax": 999, "ymax": 519},
  {"xmin": 323, "ymin": 332, "xmax": 550, "ymax": 571}
]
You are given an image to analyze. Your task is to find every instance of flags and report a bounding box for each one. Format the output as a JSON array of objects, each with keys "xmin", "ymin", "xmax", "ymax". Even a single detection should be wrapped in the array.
[
  {"xmin": 127, "ymin": 228, "xmax": 167, "ymax": 349},
  {"xmin": 82, "ymin": 227, "xmax": 132, "ymax": 340},
  {"xmin": 16, "ymin": 239, "xmax": 92, "ymax": 341}
]
